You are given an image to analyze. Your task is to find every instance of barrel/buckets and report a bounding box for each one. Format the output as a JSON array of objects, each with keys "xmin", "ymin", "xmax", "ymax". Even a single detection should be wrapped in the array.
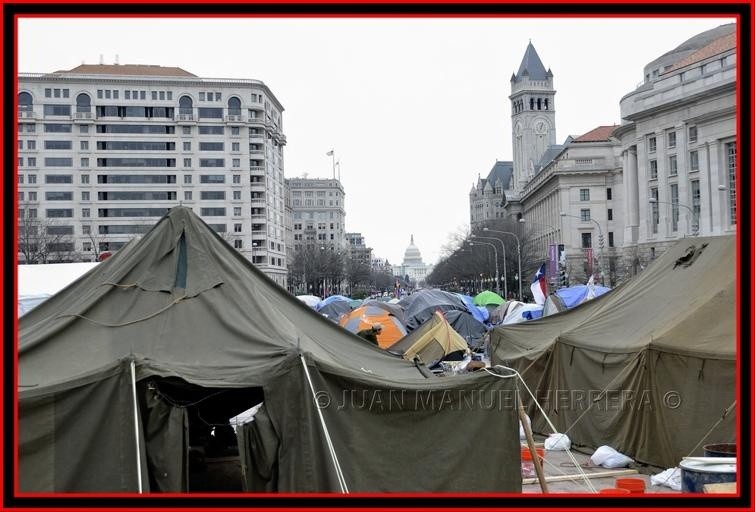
[
  {"xmin": 602, "ymin": 478, "xmax": 646, "ymax": 497},
  {"xmin": 522, "ymin": 447, "xmax": 544, "ymax": 481},
  {"xmin": 680, "ymin": 456, "xmax": 735, "ymax": 494},
  {"xmin": 703, "ymin": 441, "xmax": 735, "ymax": 455}
]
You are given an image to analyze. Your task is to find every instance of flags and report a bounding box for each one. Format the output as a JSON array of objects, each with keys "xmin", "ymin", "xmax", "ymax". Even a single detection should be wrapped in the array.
[{"xmin": 327, "ymin": 150, "xmax": 334, "ymax": 156}]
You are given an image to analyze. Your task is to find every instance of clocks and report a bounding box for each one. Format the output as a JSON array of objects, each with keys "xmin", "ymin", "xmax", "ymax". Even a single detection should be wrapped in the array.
[
  {"xmin": 514, "ymin": 119, "xmax": 524, "ymax": 136},
  {"xmin": 536, "ymin": 120, "xmax": 547, "ymax": 134}
]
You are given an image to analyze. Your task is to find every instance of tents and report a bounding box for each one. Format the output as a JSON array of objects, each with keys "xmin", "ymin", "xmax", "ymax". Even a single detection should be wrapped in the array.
[{"xmin": 16, "ymin": 203, "xmax": 736, "ymax": 495}]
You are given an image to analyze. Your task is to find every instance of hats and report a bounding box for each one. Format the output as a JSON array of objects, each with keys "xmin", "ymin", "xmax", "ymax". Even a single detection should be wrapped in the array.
[{"xmin": 372, "ymin": 323, "xmax": 384, "ymax": 330}]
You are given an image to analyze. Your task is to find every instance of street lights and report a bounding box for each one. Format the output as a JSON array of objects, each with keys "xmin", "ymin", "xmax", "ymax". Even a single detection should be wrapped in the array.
[
  {"xmin": 484, "ymin": 228, "xmax": 524, "ymax": 302},
  {"xmin": 519, "ymin": 218, "xmax": 560, "ymax": 288},
  {"xmin": 648, "ymin": 198, "xmax": 698, "ymax": 236},
  {"xmin": 560, "ymin": 212, "xmax": 606, "ymax": 287},
  {"xmin": 467, "ymin": 240, "xmax": 499, "ymax": 295},
  {"xmin": 471, "ymin": 234, "xmax": 508, "ymax": 301}
]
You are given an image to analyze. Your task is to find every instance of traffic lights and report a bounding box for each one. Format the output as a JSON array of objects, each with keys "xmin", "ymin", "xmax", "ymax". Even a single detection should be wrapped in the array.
[{"xmin": 560, "ymin": 271, "xmax": 567, "ymax": 286}]
